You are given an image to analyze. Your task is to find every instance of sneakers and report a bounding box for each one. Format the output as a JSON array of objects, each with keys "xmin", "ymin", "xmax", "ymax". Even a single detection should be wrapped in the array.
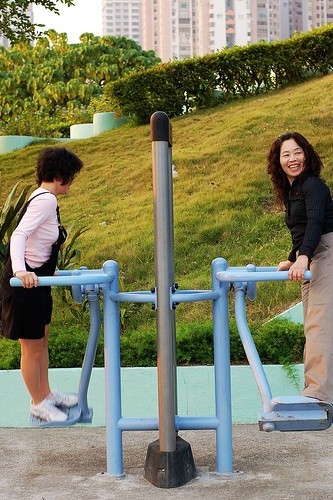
[{"xmin": 29, "ymin": 391, "xmax": 78, "ymax": 423}]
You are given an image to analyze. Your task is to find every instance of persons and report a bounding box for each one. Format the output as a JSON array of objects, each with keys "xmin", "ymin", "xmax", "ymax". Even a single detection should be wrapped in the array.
[
  {"xmin": 0, "ymin": 147, "xmax": 83, "ymax": 422},
  {"xmin": 266, "ymin": 131, "xmax": 333, "ymax": 405}
]
